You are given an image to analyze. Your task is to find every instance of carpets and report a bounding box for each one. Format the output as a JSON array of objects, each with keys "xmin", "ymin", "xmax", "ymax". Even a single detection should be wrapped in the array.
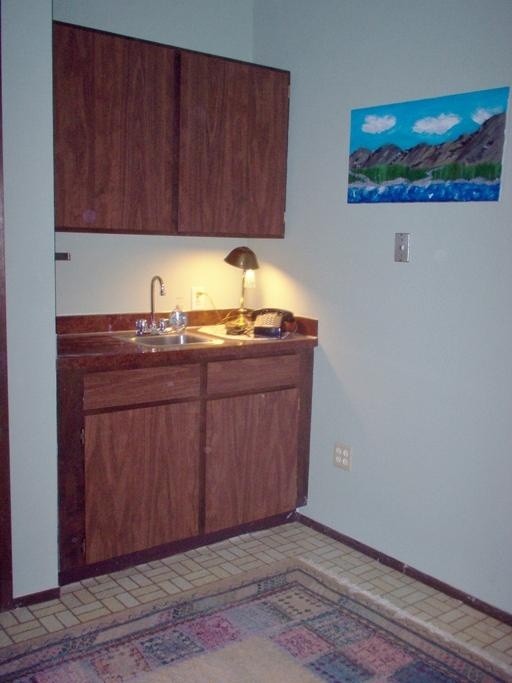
[{"xmin": 0, "ymin": 555, "xmax": 511, "ymax": 683}]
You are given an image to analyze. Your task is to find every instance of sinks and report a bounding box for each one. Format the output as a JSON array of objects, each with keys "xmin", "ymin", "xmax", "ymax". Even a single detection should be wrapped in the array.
[{"xmin": 126, "ymin": 331, "xmax": 223, "ymax": 347}]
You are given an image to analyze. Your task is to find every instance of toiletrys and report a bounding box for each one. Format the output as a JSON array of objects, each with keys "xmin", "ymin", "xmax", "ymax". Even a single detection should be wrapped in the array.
[{"xmin": 168, "ymin": 302, "xmax": 188, "ymax": 331}]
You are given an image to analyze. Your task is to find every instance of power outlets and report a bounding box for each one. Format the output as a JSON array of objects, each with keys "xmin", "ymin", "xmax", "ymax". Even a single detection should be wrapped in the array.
[{"xmin": 190, "ymin": 285, "xmax": 210, "ymax": 310}]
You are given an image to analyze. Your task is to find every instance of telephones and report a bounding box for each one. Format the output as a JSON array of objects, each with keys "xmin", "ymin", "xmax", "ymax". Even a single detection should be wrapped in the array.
[{"xmin": 252, "ymin": 308, "xmax": 295, "ymax": 337}]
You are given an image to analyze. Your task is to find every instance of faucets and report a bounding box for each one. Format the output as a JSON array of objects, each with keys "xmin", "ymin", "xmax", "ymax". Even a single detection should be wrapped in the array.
[{"xmin": 147, "ymin": 275, "xmax": 167, "ymax": 335}]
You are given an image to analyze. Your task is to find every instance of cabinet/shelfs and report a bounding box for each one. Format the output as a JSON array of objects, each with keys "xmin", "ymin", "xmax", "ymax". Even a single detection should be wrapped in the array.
[
  {"xmin": 52, "ymin": 19, "xmax": 291, "ymax": 239},
  {"xmin": 82, "ymin": 353, "xmax": 303, "ymax": 567}
]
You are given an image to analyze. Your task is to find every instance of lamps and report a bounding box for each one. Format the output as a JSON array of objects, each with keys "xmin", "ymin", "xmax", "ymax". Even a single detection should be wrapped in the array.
[{"xmin": 224, "ymin": 246, "xmax": 260, "ymax": 335}]
